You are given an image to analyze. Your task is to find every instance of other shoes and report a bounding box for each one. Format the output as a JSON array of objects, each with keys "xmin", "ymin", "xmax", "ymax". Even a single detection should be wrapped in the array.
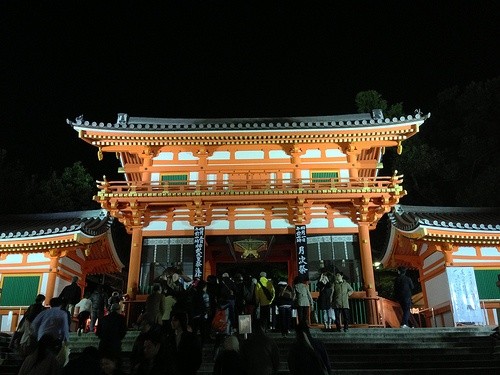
[
  {"xmin": 343, "ymin": 328, "xmax": 349, "ymax": 332},
  {"xmin": 339, "ymin": 329, "xmax": 341, "ymax": 332},
  {"xmin": 401, "ymin": 324, "xmax": 410, "ymax": 328}
]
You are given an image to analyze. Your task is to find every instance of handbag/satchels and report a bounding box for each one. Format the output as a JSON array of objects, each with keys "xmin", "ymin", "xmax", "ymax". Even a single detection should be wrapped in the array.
[
  {"xmin": 328, "ymin": 306, "xmax": 335, "ymax": 320},
  {"xmin": 259, "ymin": 280, "xmax": 272, "ymax": 301},
  {"xmin": 55, "ymin": 342, "xmax": 71, "ymax": 367}
]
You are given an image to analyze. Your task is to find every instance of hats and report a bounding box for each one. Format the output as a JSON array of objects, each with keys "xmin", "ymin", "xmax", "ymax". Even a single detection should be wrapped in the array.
[
  {"xmin": 222, "ymin": 273, "xmax": 229, "ymax": 278},
  {"xmin": 260, "ymin": 272, "xmax": 266, "ymax": 277},
  {"xmin": 137, "ymin": 312, "xmax": 154, "ymax": 325}
]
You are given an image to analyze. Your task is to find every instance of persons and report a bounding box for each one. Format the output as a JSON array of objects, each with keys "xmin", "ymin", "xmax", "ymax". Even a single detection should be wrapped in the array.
[
  {"xmin": 394, "ymin": 267, "xmax": 416, "ymax": 328},
  {"xmin": 16, "ymin": 267, "xmax": 354, "ymax": 375}
]
